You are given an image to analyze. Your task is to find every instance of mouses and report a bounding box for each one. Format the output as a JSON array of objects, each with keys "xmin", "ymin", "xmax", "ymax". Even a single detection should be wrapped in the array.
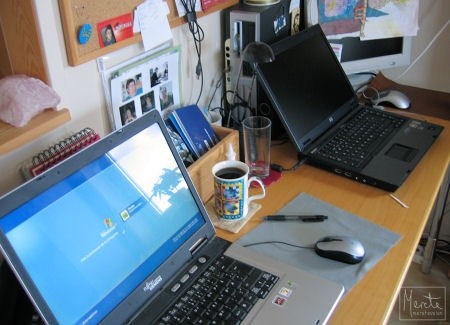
[
  {"xmin": 371, "ymin": 91, "xmax": 411, "ymax": 110},
  {"xmin": 315, "ymin": 236, "xmax": 365, "ymax": 263}
]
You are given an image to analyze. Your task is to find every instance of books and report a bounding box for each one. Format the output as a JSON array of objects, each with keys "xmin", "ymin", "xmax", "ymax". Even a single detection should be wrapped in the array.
[
  {"xmin": 17, "ymin": 127, "xmax": 101, "ymax": 183},
  {"xmin": 168, "ymin": 104, "xmax": 220, "ymax": 160}
]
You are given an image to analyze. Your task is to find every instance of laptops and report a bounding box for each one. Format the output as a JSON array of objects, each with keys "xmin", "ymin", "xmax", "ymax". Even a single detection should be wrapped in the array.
[
  {"xmin": 252, "ymin": 24, "xmax": 444, "ymax": 194},
  {"xmin": 0, "ymin": 110, "xmax": 345, "ymax": 325}
]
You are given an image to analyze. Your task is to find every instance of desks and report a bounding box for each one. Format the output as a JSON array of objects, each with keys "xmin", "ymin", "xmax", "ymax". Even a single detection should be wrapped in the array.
[{"xmin": 1, "ymin": 75, "xmax": 450, "ymax": 325}]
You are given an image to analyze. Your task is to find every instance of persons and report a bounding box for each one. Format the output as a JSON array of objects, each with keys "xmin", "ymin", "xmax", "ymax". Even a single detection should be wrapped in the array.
[
  {"xmin": 124, "ymin": 108, "xmax": 135, "ymax": 124},
  {"xmin": 151, "ymin": 67, "xmax": 162, "ymax": 88},
  {"xmin": 143, "ymin": 96, "xmax": 154, "ymax": 112},
  {"xmin": 160, "ymin": 87, "xmax": 173, "ymax": 109},
  {"xmin": 103, "ymin": 27, "xmax": 115, "ymax": 46},
  {"xmin": 125, "ymin": 79, "xmax": 143, "ymax": 101}
]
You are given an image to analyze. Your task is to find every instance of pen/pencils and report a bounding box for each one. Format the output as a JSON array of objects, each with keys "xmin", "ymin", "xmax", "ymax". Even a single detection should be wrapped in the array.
[{"xmin": 258, "ymin": 215, "xmax": 328, "ymax": 223}]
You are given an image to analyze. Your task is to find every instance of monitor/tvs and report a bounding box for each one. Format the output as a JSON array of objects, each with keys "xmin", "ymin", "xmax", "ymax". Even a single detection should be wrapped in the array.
[{"xmin": 300, "ymin": 0, "xmax": 412, "ymax": 75}]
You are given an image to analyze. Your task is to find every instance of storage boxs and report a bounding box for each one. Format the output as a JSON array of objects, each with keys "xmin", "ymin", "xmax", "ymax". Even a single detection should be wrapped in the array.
[{"xmin": 185, "ymin": 122, "xmax": 240, "ymax": 206}]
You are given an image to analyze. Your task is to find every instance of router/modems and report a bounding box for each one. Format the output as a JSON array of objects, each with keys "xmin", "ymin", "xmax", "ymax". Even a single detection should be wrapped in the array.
[{"xmin": 224, "ymin": 39, "xmax": 231, "ymax": 105}]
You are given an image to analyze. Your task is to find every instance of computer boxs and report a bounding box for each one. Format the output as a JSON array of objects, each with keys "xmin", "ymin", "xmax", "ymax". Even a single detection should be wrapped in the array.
[{"xmin": 230, "ymin": 0, "xmax": 290, "ymax": 141}]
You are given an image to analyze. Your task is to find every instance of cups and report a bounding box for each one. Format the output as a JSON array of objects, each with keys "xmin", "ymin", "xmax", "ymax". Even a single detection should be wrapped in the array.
[
  {"xmin": 212, "ymin": 160, "xmax": 265, "ymax": 222},
  {"xmin": 241, "ymin": 116, "xmax": 271, "ymax": 178}
]
